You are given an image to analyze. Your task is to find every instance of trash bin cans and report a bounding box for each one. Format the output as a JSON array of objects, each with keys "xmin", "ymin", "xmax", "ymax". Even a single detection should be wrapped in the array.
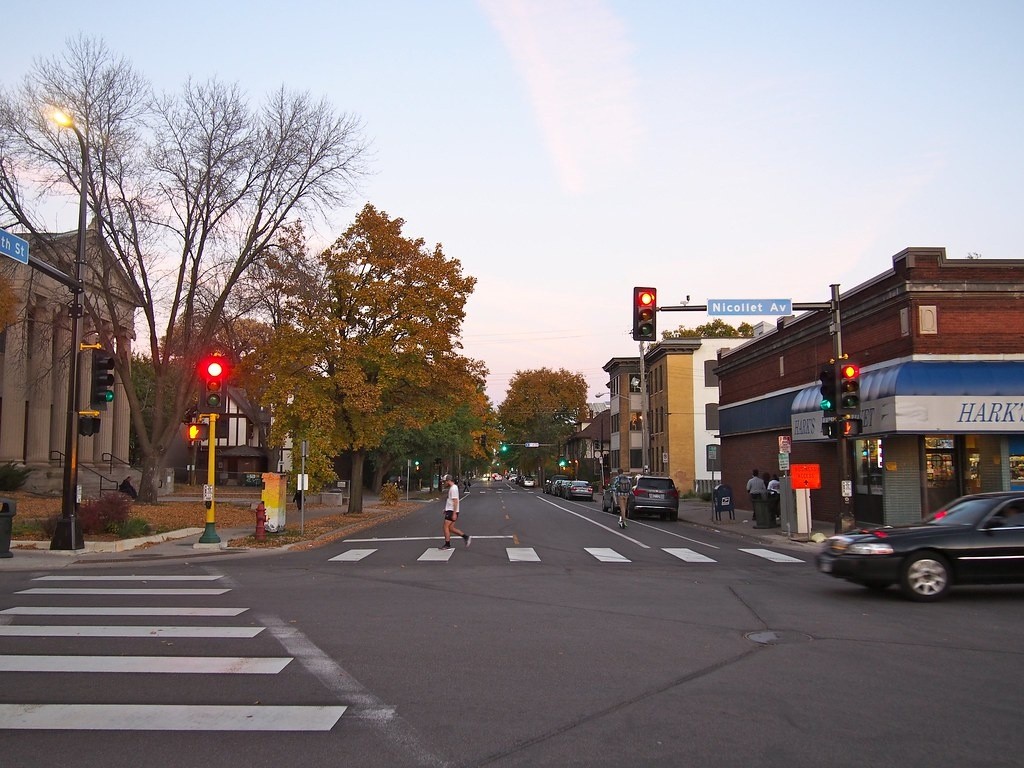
[
  {"xmin": 0, "ymin": 498, "xmax": 16, "ymax": 558},
  {"xmin": 713, "ymin": 484, "xmax": 735, "ymax": 521},
  {"xmin": 753, "ymin": 496, "xmax": 778, "ymax": 528}
]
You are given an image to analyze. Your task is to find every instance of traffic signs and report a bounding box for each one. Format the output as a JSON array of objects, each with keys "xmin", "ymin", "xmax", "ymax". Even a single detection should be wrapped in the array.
[{"xmin": 790, "ymin": 463, "xmax": 821, "ymax": 491}]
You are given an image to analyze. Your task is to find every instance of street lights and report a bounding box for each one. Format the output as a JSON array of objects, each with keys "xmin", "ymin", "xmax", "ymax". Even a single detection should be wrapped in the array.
[
  {"xmin": 48, "ymin": 106, "xmax": 89, "ymax": 552},
  {"xmin": 596, "ymin": 391, "xmax": 651, "ymax": 476}
]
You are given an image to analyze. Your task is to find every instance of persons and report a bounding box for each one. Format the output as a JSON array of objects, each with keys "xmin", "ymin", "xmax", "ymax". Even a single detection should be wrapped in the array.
[
  {"xmin": 746, "ymin": 469, "xmax": 781, "ymax": 520},
  {"xmin": 611, "ymin": 469, "xmax": 633, "ymax": 528},
  {"xmin": 438, "ymin": 474, "xmax": 472, "ymax": 550},
  {"xmin": 463, "ymin": 476, "xmax": 471, "ymax": 493},
  {"xmin": 119, "ymin": 476, "xmax": 137, "ymax": 498},
  {"xmin": 454, "ymin": 475, "xmax": 459, "ymax": 484},
  {"xmin": 293, "ymin": 490, "xmax": 306, "ymax": 511},
  {"xmin": 396, "ymin": 476, "xmax": 403, "ymax": 492}
]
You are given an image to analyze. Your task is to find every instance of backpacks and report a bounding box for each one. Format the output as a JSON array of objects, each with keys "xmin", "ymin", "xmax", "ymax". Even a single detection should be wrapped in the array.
[{"xmin": 617, "ymin": 475, "xmax": 629, "ymax": 493}]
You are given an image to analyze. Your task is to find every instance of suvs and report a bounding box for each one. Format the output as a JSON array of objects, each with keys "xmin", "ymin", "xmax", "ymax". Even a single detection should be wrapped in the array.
[{"xmin": 626, "ymin": 474, "xmax": 680, "ymax": 522}]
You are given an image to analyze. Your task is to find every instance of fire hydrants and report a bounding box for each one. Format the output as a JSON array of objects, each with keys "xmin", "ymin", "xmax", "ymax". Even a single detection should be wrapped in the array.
[{"xmin": 255, "ymin": 501, "xmax": 269, "ymax": 540}]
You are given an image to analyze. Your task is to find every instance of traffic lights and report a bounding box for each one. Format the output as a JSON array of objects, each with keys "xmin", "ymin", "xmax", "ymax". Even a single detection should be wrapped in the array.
[
  {"xmin": 821, "ymin": 421, "xmax": 838, "ymax": 439},
  {"xmin": 412, "ymin": 459, "xmax": 420, "ymax": 467},
  {"xmin": 560, "ymin": 461, "xmax": 566, "ymax": 466},
  {"xmin": 81, "ymin": 416, "xmax": 101, "ymax": 437},
  {"xmin": 186, "ymin": 422, "xmax": 211, "ymax": 441},
  {"xmin": 841, "ymin": 418, "xmax": 864, "ymax": 437},
  {"xmin": 501, "ymin": 446, "xmax": 507, "ymax": 451},
  {"xmin": 836, "ymin": 364, "xmax": 861, "ymax": 415},
  {"xmin": 91, "ymin": 347, "xmax": 114, "ymax": 410},
  {"xmin": 820, "ymin": 364, "xmax": 835, "ymax": 410},
  {"xmin": 198, "ymin": 357, "xmax": 229, "ymax": 414},
  {"xmin": 633, "ymin": 287, "xmax": 657, "ymax": 340}
]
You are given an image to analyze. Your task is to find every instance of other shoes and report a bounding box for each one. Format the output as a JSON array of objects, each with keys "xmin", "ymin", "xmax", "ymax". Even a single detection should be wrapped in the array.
[
  {"xmin": 439, "ymin": 546, "xmax": 451, "ymax": 551},
  {"xmin": 618, "ymin": 521, "xmax": 627, "ymax": 529},
  {"xmin": 465, "ymin": 536, "xmax": 471, "ymax": 549}
]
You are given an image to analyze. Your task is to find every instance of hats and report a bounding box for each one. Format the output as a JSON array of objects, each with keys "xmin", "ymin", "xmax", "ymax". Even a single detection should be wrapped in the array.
[{"xmin": 441, "ymin": 474, "xmax": 453, "ymax": 481}]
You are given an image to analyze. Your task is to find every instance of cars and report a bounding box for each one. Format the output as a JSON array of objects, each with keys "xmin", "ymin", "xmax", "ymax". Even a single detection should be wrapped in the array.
[
  {"xmin": 544, "ymin": 474, "xmax": 594, "ymax": 502},
  {"xmin": 601, "ymin": 476, "xmax": 632, "ymax": 514},
  {"xmin": 491, "ymin": 472, "xmax": 535, "ymax": 488},
  {"xmin": 814, "ymin": 490, "xmax": 1024, "ymax": 603}
]
